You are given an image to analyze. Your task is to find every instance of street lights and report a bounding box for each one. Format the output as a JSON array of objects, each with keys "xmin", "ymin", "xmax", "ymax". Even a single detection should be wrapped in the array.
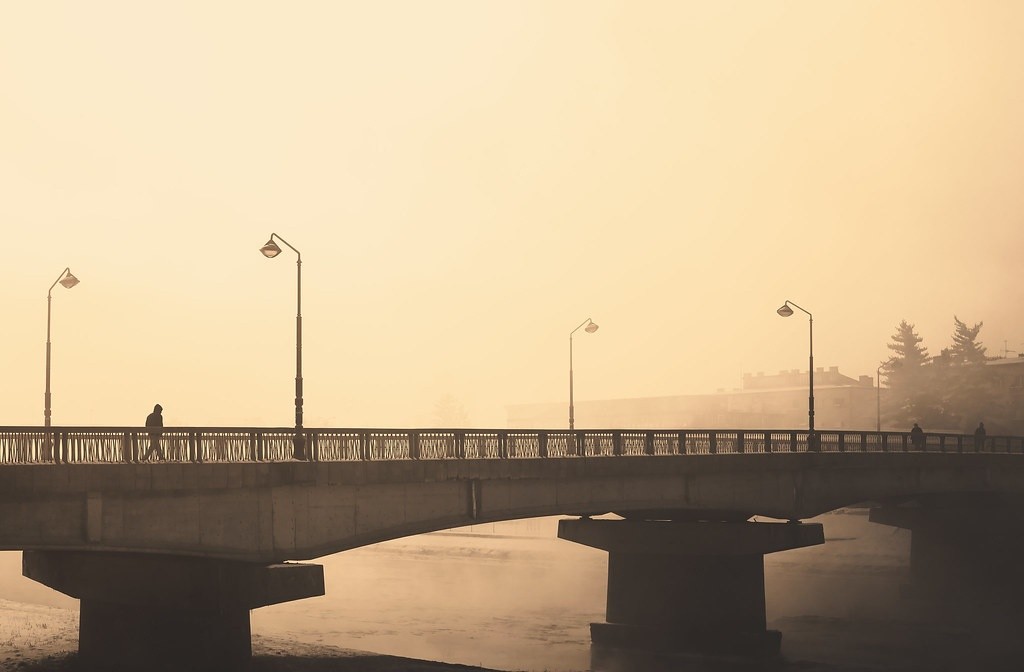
[
  {"xmin": 777, "ymin": 301, "xmax": 817, "ymax": 451},
  {"xmin": 258, "ymin": 233, "xmax": 309, "ymax": 461},
  {"xmin": 566, "ymin": 317, "xmax": 599, "ymax": 455},
  {"xmin": 877, "ymin": 356, "xmax": 902, "ymax": 450},
  {"xmin": 42, "ymin": 267, "xmax": 82, "ymax": 461}
]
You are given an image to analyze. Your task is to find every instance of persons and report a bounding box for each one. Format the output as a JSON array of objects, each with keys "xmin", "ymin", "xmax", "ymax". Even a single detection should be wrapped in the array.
[
  {"xmin": 142, "ymin": 404, "xmax": 166, "ymax": 461},
  {"xmin": 910, "ymin": 423, "xmax": 923, "ymax": 449},
  {"xmin": 975, "ymin": 422, "xmax": 986, "ymax": 452}
]
